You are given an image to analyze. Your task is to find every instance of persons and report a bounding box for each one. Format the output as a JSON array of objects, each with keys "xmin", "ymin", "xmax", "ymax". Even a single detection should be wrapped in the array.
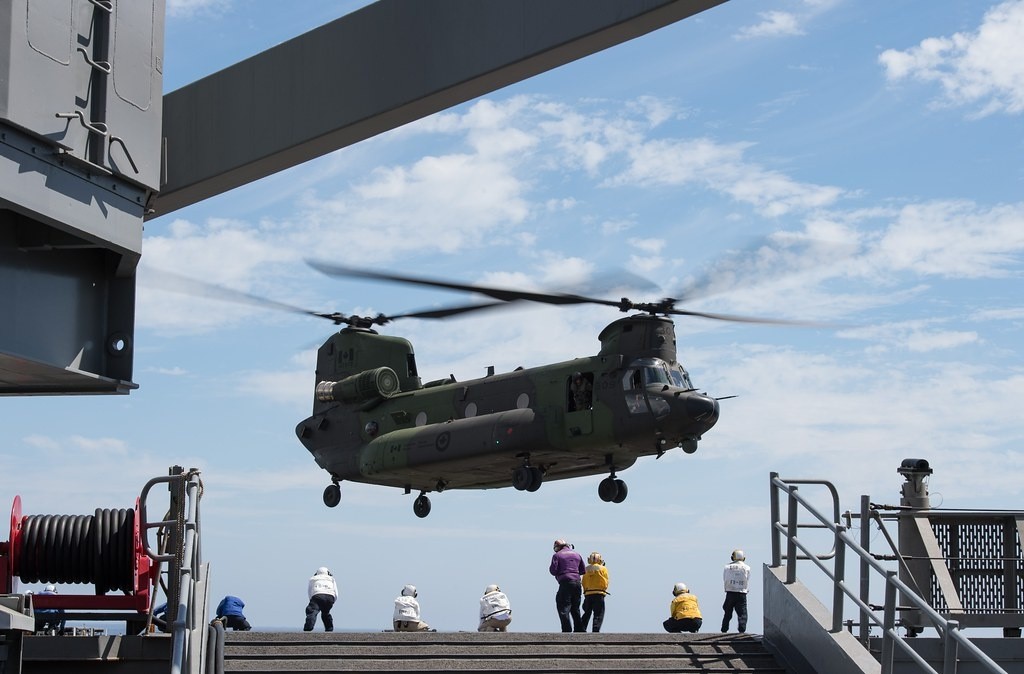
[
  {"xmin": 477, "ymin": 585, "xmax": 512, "ymax": 632},
  {"xmin": 663, "ymin": 583, "xmax": 703, "ymax": 633},
  {"xmin": 393, "ymin": 585, "xmax": 430, "ymax": 631},
  {"xmin": 215, "ymin": 596, "xmax": 250, "ymax": 631},
  {"xmin": 721, "ymin": 550, "xmax": 750, "ymax": 633},
  {"xmin": 581, "ymin": 553, "xmax": 610, "ymax": 633},
  {"xmin": 304, "ymin": 567, "xmax": 338, "ymax": 631},
  {"xmin": 24, "ymin": 585, "xmax": 66, "ymax": 636},
  {"xmin": 549, "ymin": 539, "xmax": 586, "ymax": 633},
  {"xmin": 153, "ymin": 603, "xmax": 169, "ymax": 632}
]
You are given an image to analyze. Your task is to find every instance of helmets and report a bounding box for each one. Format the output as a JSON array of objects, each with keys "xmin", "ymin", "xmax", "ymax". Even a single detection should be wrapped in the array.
[
  {"xmin": 401, "ymin": 585, "xmax": 417, "ymax": 598},
  {"xmin": 588, "ymin": 552, "xmax": 604, "ymax": 564},
  {"xmin": 673, "ymin": 583, "xmax": 689, "ymax": 597},
  {"xmin": 554, "ymin": 539, "xmax": 566, "ymax": 553},
  {"xmin": 316, "ymin": 567, "xmax": 328, "ymax": 576},
  {"xmin": 731, "ymin": 549, "xmax": 746, "ymax": 561},
  {"xmin": 485, "ymin": 585, "xmax": 501, "ymax": 595}
]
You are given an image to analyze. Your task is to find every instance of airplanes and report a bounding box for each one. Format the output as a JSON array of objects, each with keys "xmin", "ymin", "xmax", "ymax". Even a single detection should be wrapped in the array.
[{"xmin": 145, "ymin": 255, "xmax": 867, "ymax": 520}]
